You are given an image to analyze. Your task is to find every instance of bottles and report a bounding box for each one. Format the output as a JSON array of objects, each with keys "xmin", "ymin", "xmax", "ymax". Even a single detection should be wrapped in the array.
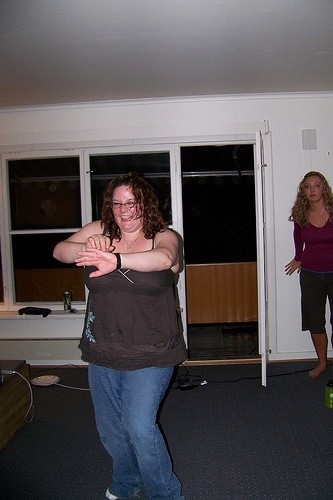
[{"xmin": 326, "ymin": 380, "xmax": 333, "ymax": 409}]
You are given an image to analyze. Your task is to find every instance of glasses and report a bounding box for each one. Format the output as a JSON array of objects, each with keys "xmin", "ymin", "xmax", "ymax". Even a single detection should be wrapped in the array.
[{"xmin": 109, "ymin": 202, "xmax": 137, "ymax": 209}]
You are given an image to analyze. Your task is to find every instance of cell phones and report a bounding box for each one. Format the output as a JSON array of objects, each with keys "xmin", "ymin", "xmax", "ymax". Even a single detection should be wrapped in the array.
[{"xmin": 0, "ymin": 370, "xmax": 13, "ymax": 374}]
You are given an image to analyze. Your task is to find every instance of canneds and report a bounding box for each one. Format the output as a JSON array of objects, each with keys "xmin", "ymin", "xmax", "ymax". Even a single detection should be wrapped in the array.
[{"xmin": 63, "ymin": 292, "xmax": 71, "ymax": 311}]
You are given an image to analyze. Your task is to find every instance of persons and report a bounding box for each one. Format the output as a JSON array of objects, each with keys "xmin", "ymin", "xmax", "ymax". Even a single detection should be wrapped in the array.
[
  {"xmin": 53, "ymin": 172, "xmax": 189, "ymax": 500},
  {"xmin": 285, "ymin": 171, "xmax": 333, "ymax": 378}
]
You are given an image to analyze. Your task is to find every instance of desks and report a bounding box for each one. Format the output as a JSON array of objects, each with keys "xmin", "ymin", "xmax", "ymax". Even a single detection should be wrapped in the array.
[{"xmin": 0, "ymin": 360, "xmax": 31, "ymax": 452}]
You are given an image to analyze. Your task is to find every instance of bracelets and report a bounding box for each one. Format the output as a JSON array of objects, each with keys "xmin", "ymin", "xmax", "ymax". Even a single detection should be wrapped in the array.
[{"xmin": 114, "ymin": 253, "xmax": 121, "ymax": 270}]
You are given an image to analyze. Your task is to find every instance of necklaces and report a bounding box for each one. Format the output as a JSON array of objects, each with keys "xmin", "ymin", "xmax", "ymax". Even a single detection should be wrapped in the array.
[{"xmin": 121, "ymin": 232, "xmax": 142, "ymax": 249}]
